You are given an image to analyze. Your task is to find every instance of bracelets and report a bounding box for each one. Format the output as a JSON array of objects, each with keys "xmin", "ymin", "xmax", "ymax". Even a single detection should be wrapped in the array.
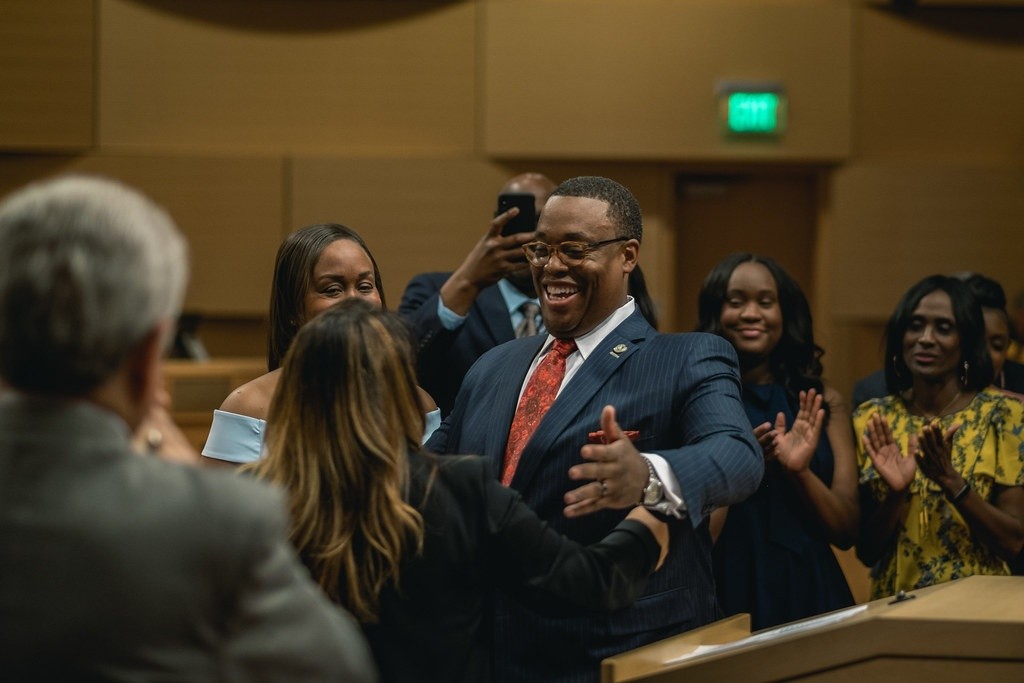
[{"xmin": 945, "ymin": 478, "xmax": 969, "ymax": 503}]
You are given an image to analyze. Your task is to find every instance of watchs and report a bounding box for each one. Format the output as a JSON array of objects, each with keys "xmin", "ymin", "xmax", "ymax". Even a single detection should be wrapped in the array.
[{"xmin": 642, "ymin": 458, "xmax": 664, "ymax": 505}]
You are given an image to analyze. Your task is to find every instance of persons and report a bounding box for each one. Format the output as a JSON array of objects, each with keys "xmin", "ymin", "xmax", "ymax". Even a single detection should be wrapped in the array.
[
  {"xmin": 694, "ymin": 253, "xmax": 859, "ymax": 630},
  {"xmin": 0, "ymin": 174, "xmax": 376, "ymax": 683},
  {"xmin": 855, "ymin": 272, "xmax": 1024, "ymax": 598},
  {"xmin": 398, "ymin": 172, "xmax": 657, "ymax": 417},
  {"xmin": 200, "ymin": 223, "xmax": 441, "ymax": 480},
  {"xmin": 418, "ymin": 177, "xmax": 764, "ymax": 683},
  {"xmin": 234, "ymin": 296, "xmax": 671, "ymax": 683}
]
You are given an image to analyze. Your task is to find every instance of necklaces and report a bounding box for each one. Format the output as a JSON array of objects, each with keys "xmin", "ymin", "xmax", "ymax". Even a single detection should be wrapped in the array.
[{"xmin": 912, "ymin": 392, "xmax": 961, "ymax": 418}]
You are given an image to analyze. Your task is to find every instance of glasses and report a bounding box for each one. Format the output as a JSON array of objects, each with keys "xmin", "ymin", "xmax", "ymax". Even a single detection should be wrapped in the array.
[{"xmin": 522, "ymin": 236, "xmax": 630, "ymax": 268}]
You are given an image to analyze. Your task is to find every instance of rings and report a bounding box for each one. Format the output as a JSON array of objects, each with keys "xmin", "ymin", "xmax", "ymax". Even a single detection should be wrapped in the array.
[{"xmin": 600, "ymin": 481, "xmax": 607, "ymax": 495}]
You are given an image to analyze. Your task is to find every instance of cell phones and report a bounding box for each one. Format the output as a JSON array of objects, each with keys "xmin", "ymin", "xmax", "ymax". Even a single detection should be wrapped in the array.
[{"xmin": 498, "ymin": 192, "xmax": 537, "ymax": 263}]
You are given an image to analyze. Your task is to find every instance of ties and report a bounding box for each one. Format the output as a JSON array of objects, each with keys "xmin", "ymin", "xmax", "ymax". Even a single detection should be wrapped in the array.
[{"xmin": 501, "ymin": 339, "xmax": 578, "ymax": 488}]
